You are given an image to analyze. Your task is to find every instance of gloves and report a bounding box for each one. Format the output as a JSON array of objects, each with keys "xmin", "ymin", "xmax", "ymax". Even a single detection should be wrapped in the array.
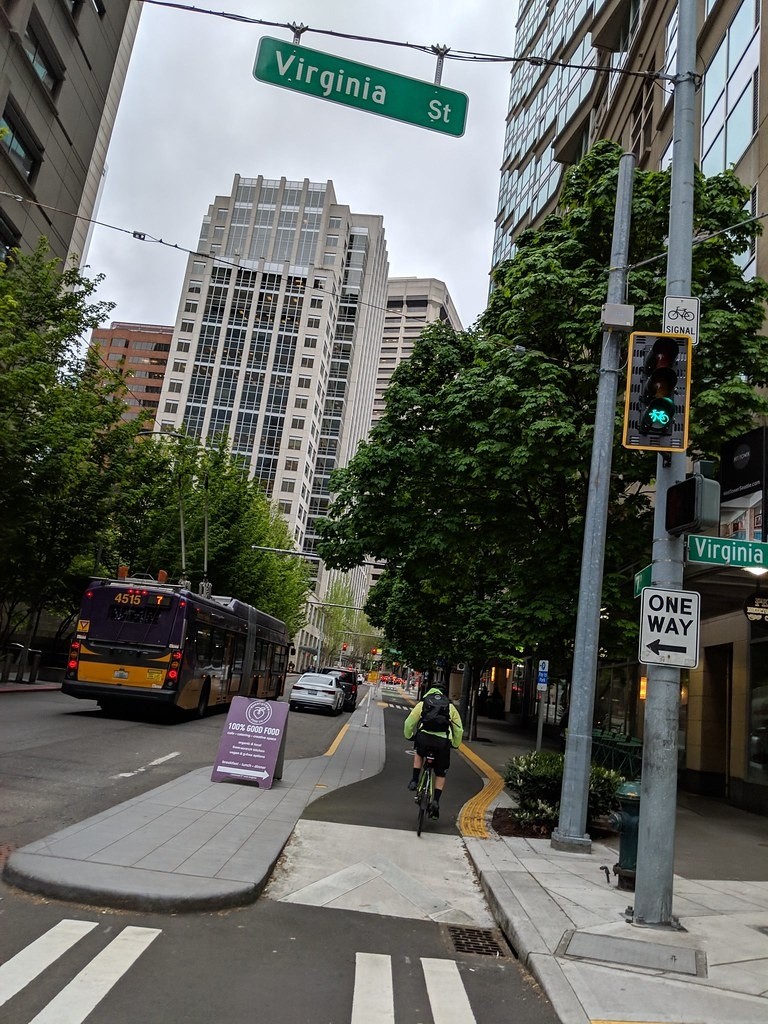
[
  {"xmin": 450, "ymin": 742, "xmax": 458, "ymax": 749},
  {"xmin": 408, "ymin": 734, "xmax": 415, "ymax": 741}
]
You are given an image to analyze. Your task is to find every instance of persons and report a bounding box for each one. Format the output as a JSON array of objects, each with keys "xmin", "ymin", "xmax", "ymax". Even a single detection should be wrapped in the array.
[
  {"xmin": 288, "ymin": 662, "xmax": 295, "ymax": 673},
  {"xmin": 403, "ymin": 681, "xmax": 463, "ymax": 820}
]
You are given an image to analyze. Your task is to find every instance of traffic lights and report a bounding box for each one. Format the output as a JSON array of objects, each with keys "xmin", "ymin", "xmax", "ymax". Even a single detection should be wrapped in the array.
[
  {"xmin": 624, "ymin": 330, "xmax": 692, "ymax": 452},
  {"xmin": 343, "ymin": 643, "xmax": 347, "ymax": 651},
  {"xmin": 372, "ymin": 648, "xmax": 377, "ymax": 655},
  {"xmin": 666, "ymin": 477, "xmax": 720, "ymax": 537}
]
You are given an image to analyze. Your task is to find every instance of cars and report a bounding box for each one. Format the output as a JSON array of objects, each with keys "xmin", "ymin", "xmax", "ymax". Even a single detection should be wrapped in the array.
[
  {"xmin": 6, "ymin": 642, "xmax": 42, "ymax": 666},
  {"xmin": 289, "ymin": 672, "xmax": 346, "ymax": 717},
  {"xmin": 357, "ymin": 674, "xmax": 363, "ymax": 684}
]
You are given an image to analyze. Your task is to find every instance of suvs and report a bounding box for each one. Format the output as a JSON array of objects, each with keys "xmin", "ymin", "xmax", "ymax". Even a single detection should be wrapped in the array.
[{"xmin": 321, "ymin": 667, "xmax": 358, "ymax": 712}]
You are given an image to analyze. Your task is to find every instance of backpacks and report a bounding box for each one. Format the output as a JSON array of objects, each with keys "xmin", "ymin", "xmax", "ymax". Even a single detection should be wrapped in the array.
[{"xmin": 417, "ymin": 693, "xmax": 450, "ymax": 731}]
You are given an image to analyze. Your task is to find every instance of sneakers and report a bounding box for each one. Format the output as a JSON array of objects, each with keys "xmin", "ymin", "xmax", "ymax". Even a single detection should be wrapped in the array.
[
  {"xmin": 429, "ymin": 800, "xmax": 440, "ymax": 820},
  {"xmin": 408, "ymin": 780, "xmax": 417, "ymax": 791}
]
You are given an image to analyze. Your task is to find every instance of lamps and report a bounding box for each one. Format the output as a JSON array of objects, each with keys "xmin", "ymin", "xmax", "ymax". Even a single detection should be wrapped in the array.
[{"xmin": 639, "ymin": 677, "xmax": 647, "ymax": 701}]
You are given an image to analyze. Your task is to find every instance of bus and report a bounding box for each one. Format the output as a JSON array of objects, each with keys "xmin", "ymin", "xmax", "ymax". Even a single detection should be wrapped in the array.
[{"xmin": 60, "ymin": 473, "xmax": 296, "ymax": 720}]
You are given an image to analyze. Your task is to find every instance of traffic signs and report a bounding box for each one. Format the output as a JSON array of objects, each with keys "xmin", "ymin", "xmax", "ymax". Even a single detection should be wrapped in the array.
[{"xmin": 638, "ymin": 586, "xmax": 701, "ymax": 669}]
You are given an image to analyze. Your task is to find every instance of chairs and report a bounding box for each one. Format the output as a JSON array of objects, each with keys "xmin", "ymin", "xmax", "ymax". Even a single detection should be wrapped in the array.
[{"xmin": 592, "ymin": 730, "xmax": 643, "ymax": 780}]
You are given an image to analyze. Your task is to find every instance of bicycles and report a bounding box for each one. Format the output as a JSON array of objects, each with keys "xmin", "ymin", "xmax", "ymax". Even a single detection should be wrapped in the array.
[{"xmin": 412, "ymin": 737, "xmax": 459, "ymax": 837}]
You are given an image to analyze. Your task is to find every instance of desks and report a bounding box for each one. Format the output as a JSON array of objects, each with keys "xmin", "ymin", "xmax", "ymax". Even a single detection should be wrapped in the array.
[
  {"xmin": 616, "ymin": 742, "xmax": 641, "ymax": 777},
  {"xmin": 600, "ymin": 738, "xmax": 626, "ymax": 769},
  {"xmin": 592, "ymin": 732, "xmax": 601, "ymax": 763}
]
